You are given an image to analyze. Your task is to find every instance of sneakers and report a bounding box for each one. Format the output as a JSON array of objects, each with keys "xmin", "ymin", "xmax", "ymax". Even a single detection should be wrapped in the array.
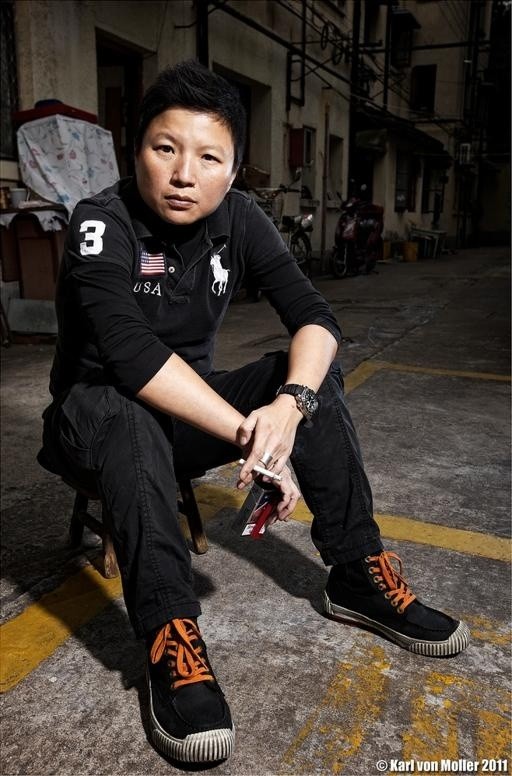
[
  {"xmin": 138, "ymin": 617, "xmax": 237, "ymax": 766},
  {"xmin": 322, "ymin": 549, "xmax": 470, "ymax": 657}
]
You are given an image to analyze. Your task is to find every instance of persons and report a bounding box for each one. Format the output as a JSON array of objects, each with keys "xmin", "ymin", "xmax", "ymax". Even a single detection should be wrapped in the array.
[{"xmin": 35, "ymin": 59, "xmax": 474, "ymax": 772}]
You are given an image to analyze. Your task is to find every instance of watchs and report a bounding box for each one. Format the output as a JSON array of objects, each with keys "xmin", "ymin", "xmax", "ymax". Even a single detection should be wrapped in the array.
[{"xmin": 275, "ymin": 383, "xmax": 322, "ymax": 420}]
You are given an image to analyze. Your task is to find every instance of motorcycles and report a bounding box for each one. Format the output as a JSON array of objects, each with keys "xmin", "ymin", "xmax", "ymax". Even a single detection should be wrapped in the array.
[
  {"xmin": 331, "ymin": 190, "xmax": 385, "ymax": 278},
  {"xmin": 240, "ymin": 166, "xmax": 314, "ymax": 280}
]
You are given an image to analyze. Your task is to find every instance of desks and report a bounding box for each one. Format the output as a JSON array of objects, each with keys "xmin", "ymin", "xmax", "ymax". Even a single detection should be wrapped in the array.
[{"xmin": 410, "ymin": 226, "xmax": 446, "ymax": 259}]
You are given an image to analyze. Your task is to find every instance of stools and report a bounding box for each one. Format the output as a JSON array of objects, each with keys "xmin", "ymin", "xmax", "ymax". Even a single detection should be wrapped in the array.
[{"xmin": 61, "ymin": 465, "xmax": 212, "ymax": 578}]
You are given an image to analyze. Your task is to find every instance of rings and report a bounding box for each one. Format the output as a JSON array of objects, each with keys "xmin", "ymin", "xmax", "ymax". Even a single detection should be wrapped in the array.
[{"xmin": 259, "ymin": 452, "xmax": 273, "ymax": 467}]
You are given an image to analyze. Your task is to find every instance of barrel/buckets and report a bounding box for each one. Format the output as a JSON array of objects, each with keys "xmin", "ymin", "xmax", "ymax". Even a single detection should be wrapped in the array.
[
  {"xmin": 382, "ymin": 239, "xmax": 391, "ymax": 259},
  {"xmin": 403, "ymin": 242, "xmax": 418, "ymax": 260}
]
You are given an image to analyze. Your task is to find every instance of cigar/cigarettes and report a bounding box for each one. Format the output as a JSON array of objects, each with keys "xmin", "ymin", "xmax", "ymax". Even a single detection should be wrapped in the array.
[{"xmin": 238, "ymin": 458, "xmax": 283, "ymax": 482}]
